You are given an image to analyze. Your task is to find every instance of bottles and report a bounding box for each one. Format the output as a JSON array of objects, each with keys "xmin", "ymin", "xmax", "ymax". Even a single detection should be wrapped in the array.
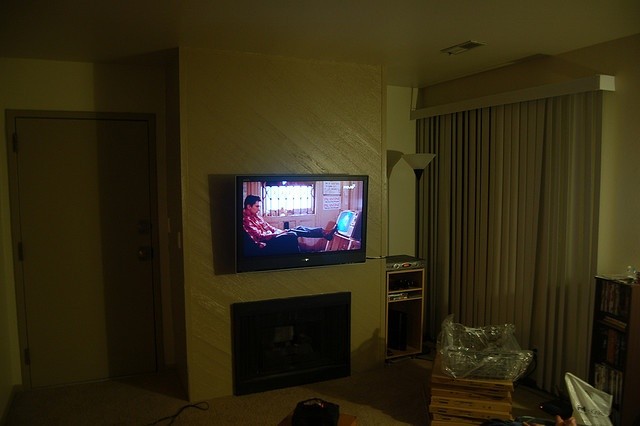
[{"xmin": 626, "ymin": 265, "xmax": 634, "ymax": 285}]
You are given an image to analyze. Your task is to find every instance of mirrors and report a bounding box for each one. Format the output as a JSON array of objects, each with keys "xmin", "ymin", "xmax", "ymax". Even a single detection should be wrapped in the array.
[{"xmin": 386, "ymin": 83, "xmax": 419, "ymax": 257}]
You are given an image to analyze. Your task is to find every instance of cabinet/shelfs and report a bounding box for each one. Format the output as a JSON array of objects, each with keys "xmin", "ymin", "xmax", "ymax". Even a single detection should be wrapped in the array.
[
  {"xmin": 588, "ymin": 274, "xmax": 640, "ymax": 424},
  {"xmin": 385, "ymin": 267, "xmax": 425, "ymax": 359}
]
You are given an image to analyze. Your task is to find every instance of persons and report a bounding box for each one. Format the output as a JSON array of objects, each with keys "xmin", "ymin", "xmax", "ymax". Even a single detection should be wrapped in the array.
[{"xmin": 243, "ymin": 194, "xmax": 338, "ymax": 252}]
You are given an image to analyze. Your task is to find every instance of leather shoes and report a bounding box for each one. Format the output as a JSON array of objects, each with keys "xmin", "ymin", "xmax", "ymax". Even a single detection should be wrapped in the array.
[{"xmin": 324, "ymin": 223, "xmax": 337, "ymax": 240}]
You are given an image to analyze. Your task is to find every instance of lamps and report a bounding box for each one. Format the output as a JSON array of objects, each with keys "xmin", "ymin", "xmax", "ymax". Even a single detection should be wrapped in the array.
[
  {"xmin": 401, "ymin": 152, "xmax": 436, "ymax": 258},
  {"xmin": 387, "ymin": 149, "xmax": 404, "ymax": 255}
]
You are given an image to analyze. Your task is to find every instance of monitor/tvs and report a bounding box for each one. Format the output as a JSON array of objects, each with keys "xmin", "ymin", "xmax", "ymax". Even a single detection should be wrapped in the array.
[{"xmin": 234, "ymin": 175, "xmax": 369, "ymax": 272}]
